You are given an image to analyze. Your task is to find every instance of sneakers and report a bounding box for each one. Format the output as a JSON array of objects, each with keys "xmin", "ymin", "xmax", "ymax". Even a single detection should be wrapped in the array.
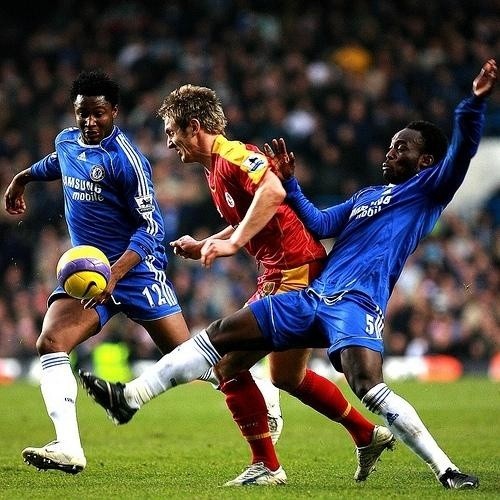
[
  {"xmin": 78, "ymin": 369, "xmax": 139, "ymax": 426},
  {"xmin": 261, "ymin": 382, "xmax": 283, "ymax": 444},
  {"xmin": 222, "ymin": 464, "xmax": 287, "ymax": 486},
  {"xmin": 355, "ymin": 425, "xmax": 395, "ymax": 481},
  {"xmin": 438, "ymin": 470, "xmax": 479, "ymax": 490},
  {"xmin": 22, "ymin": 441, "xmax": 87, "ymax": 475}
]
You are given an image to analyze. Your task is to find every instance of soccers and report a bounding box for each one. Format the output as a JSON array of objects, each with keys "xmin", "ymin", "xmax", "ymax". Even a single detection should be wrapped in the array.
[{"xmin": 57, "ymin": 245, "xmax": 110, "ymax": 299}]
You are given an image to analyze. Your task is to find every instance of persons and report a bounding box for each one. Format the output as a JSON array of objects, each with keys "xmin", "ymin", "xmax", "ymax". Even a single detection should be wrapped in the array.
[
  {"xmin": 76, "ymin": 56, "xmax": 498, "ymax": 494},
  {"xmin": 3, "ymin": 73, "xmax": 286, "ymax": 475},
  {"xmin": 0, "ymin": 0, "xmax": 499, "ymax": 386},
  {"xmin": 157, "ymin": 83, "xmax": 396, "ymax": 489}
]
